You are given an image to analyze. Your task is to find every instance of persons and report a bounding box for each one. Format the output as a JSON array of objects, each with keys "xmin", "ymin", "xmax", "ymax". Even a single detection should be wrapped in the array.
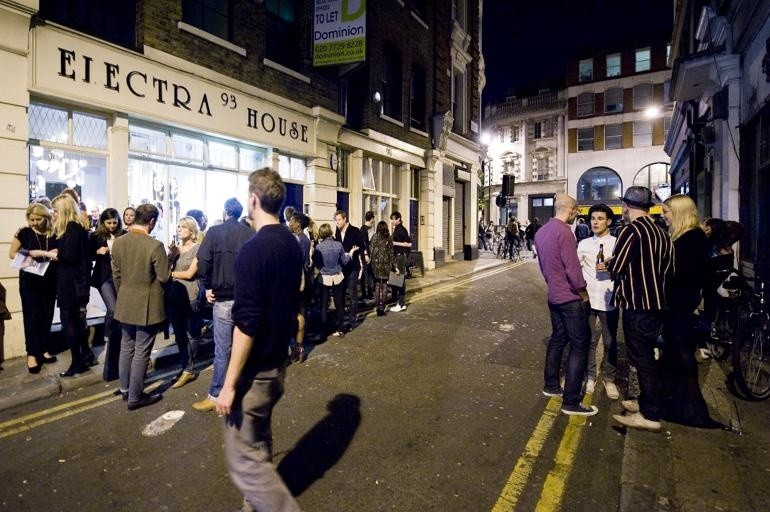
[
  {"xmin": 614, "ymin": 219, "xmax": 626, "ymax": 237},
  {"xmin": 574, "ymin": 218, "xmax": 590, "ymax": 244},
  {"xmin": 697, "ymin": 218, "xmax": 734, "ymax": 359},
  {"xmin": 213, "ymin": 166, "xmax": 309, "ymax": 510},
  {"xmin": 535, "ymin": 194, "xmax": 600, "ymax": 415},
  {"xmin": 660, "ymin": 193, "xmax": 724, "ymax": 429},
  {"xmin": 605, "ymin": 187, "xmax": 666, "ymax": 434},
  {"xmin": 577, "ymin": 203, "xmax": 623, "ymax": 400},
  {"xmin": 479, "ymin": 211, "xmax": 543, "ymax": 262}
]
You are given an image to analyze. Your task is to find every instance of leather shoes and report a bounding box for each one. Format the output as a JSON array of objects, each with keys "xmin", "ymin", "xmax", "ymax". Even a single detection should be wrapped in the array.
[
  {"xmin": 26, "ymin": 352, "xmax": 58, "ymax": 374},
  {"xmin": 620, "ymin": 398, "xmax": 640, "ymax": 413},
  {"xmin": 122, "ymin": 391, "xmax": 163, "ymax": 410},
  {"xmin": 59, "ymin": 352, "xmax": 99, "ymax": 377},
  {"xmin": 611, "ymin": 411, "xmax": 662, "ymax": 433}
]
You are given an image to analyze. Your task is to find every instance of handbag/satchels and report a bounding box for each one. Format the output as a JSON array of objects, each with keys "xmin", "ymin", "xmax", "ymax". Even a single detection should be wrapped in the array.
[{"xmin": 387, "ymin": 271, "xmax": 405, "ymax": 288}]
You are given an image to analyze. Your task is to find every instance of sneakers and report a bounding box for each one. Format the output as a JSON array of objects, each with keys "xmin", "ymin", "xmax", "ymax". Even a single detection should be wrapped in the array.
[
  {"xmin": 358, "ymin": 292, "xmax": 377, "ymax": 302},
  {"xmin": 602, "ymin": 379, "xmax": 620, "ymax": 400},
  {"xmin": 377, "ymin": 310, "xmax": 387, "ymax": 316},
  {"xmin": 389, "ymin": 303, "xmax": 407, "ymax": 313},
  {"xmin": 560, "ymin": 401, "xmax": 599, "ymax": 416},
  {"xmin": 170, "ymin": 370, "xmax": 196, "ymax": 389},
  {"xmin": 332, "ymin": 331, "xmax": 344, "ymax": 337},
  {"xmin": 542, "ymin": 386, "xmax": 565, "ymax": 397},
  {"xmin": 582, "ymin": 376, "xmax": 597, "ymax": 394},
  {"xmin": 191, "ymin": 397, "xmax": 217, "ymax": 413}
]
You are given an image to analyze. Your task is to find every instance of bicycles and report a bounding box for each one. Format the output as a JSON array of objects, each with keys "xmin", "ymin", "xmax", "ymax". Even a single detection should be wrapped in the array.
[
  {"xmin": 707, "ymin": 262, "xmax": 769, "ymax": 403},
  {"xmin": 494, "ymin": 234, "xmax": 526, "ymax": 264}
]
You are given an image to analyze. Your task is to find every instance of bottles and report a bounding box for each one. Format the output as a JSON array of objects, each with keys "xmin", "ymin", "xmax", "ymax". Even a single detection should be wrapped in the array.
[{"xmin": 596, "ymin": 243, "xmax": 605, "ymax": 264}]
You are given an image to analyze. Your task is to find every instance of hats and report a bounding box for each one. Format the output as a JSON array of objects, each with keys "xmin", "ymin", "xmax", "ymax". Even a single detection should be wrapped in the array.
[{"xmin": 618, "ymin": 186, "xmax": 656, "ymax": 208}]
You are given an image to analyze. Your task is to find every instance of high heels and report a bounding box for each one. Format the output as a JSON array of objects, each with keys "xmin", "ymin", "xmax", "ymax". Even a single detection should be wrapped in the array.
[{"xmin": 290, "ymin": 343, "xmax": 305, "ymax": 363}]
